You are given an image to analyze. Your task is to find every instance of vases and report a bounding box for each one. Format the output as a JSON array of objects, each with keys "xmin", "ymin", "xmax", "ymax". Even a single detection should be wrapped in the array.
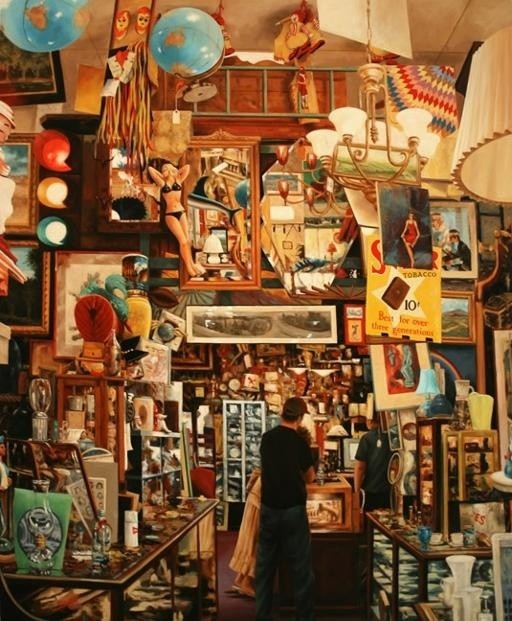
[{"xmin": 445, "ymin": 554, "xmax": 478, "ymax": 621}]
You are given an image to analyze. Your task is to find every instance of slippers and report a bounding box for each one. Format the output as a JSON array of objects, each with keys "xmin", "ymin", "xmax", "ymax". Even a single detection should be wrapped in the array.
[{"xmin": 231, "ymin": 586, "xmax": 256, "ymax": 599}]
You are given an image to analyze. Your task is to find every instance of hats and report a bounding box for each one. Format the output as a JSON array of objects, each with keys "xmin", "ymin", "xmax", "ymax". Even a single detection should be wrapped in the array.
[{"xmin": 283, "ymin": 397, "xmax": 311, "ymax": 417}]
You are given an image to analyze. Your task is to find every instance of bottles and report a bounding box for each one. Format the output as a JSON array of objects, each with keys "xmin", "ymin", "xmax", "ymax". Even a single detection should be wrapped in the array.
[
  {"xmin": 9, "ymin": 395, "xmax": 32, "ymax": 440},
  {"xmin": 123, "ymin": 510, "xmax": 139, "ymax": 549},
  {"xmin": 316, "ymin": 467, "xmax": 324, "ymax": 485},
  {"xmin": 32, "ymin": 412, "xmax": 48, "ymax": 442},
  {"xmin": 91, "ymin": 511, "xmax": 111, "ymax": 566}
]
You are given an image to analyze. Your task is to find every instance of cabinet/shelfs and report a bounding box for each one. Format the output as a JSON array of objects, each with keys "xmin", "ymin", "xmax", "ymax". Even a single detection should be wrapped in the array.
[
  {"xmin": 363, "ymin": 505, "xmax": 495, "ymax": 620},
  {"xmin": 1, "ymin": 497, "xmax": 222, "ymax": 621}
]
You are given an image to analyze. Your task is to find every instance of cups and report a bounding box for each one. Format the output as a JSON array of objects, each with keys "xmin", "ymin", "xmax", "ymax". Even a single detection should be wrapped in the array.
[
  {"xmin": 30, "ymin": 379, "xmax": 52, "ymax": 414},
  {"xmin": 417, "ymin": 527, "xmax": 431, "ymax": 552},
  {"xmin": 463, "ymin": 528, "xmax": 474, "ymax": 548},
  {"xmin": 454, "ymin": 380, "xmax": 474, "ymax": 398},
  {"xmin": 449, "ymin": 534, "xmax": 463, "ymax": 545},
  {"xmin": 318, "ymin": 390, "xmax": 348, "ymax": 415},
  {"xmin": 465, "ymin": 394, "xmax": 494, "ymax": 432},
  {"xmin": 428, "ymin": 532, "xmax": 442, "ymax": 543},
  {"xmin": 440, "ymin": 555, "xmax": 494, "ymax": 621}
]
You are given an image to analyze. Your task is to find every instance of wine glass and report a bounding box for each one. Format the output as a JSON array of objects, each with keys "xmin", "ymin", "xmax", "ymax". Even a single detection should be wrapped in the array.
[{"xmin": 29, "ymin": 479, "xmax": 55, "ymax": 576}]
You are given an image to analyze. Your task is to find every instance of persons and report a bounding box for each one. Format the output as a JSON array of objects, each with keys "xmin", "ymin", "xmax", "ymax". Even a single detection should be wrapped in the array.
[
  {"xmin": 0, "ymin": 434, "xmax": 15, "ymax": 540},
  {"xmin": 442, "ymin": 227, "xmax": 472, "ymax": 271},
  {"xmin": 353, "ymin": 410, "xmax": 398, "ymax": 534},
  {"xmin": 147, "ymin": 165, "xmax": 206, "ymax": 277},
  {"xmin": 429, "ymin": 213, "xmax": 448, "ymax": 247},
  {"xmin": 253, "ymin": 396, "xmax": 318, "ymax": 620},
  {"xmin": 226, "ymin": 424, "xmax": 313, "ymax": 600},
  {"xmin": 400, "ymin": 208, "xmax": 421, "ymax": 269}
]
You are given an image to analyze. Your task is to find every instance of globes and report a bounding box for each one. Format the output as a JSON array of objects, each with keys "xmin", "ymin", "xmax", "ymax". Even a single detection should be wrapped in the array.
[
  {"xmin": 149, "ymin": 8, "xmax": 226, "ymax": 103},
  {"xmin": 0, "ymin": 0, "xmax": 91, "ymax": 53}
]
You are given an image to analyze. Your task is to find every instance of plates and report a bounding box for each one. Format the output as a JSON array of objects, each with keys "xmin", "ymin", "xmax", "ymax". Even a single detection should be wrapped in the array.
[
  {"xmin": 448, "ymin": 543, "xmax": 463, "ymax": 548},
  {"xmin": 429, "ymin": 541, "xmax": 443, "ymax": 546},
  {"xmin": 402, "ymin": 423, "xmax": 416, "ymax": 441}
]
